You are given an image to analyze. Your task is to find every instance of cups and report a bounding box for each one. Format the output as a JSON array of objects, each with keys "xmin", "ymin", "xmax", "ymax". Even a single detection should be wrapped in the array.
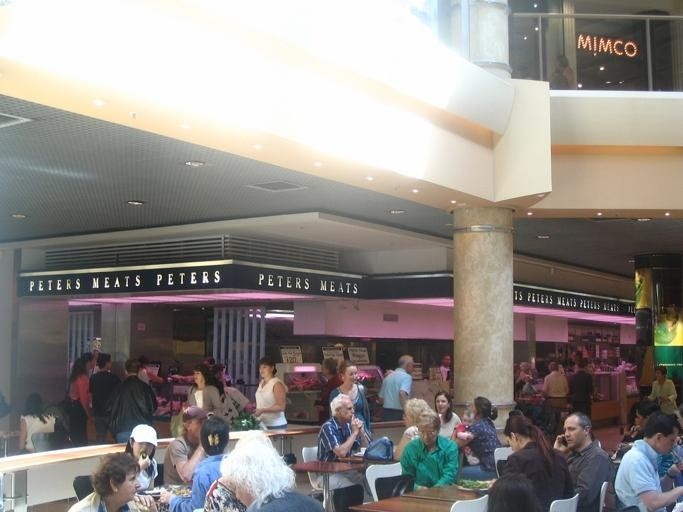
[{"xmin": 360, "ymin": 447, "xmax": 366, "ymax": 453}]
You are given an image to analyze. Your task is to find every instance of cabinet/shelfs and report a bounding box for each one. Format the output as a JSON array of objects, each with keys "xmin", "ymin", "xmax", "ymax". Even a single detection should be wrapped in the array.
[{"xmin": 285, "ymin": 365, "xmax": 384, "ymax": 422}]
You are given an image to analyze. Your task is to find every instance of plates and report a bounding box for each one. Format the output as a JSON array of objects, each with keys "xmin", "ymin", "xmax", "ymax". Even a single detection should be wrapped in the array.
[
  {"xmin": 458, "ymin": 486, "xmax": 488, "ymax": 491},
  {"xmin": 135, "ymin": 492, "xmax": 161, "ymax": 498}
]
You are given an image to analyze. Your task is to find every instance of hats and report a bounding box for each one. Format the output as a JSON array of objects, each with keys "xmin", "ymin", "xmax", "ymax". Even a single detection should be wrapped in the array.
[
  {"xmin": 130, "ymin": 424, "xmax": 158, "ymax": 447},
  {"xmin": 183, "ymin": 405, "xmax": 208, "ymax": 422}
]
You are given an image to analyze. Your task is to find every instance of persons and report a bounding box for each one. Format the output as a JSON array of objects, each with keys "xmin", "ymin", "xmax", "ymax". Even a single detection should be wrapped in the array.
[
  {"xmin": 0, "ymin": 353, "xmax": 683, "ymax": 512},
  {"xmin": 549, "ymin": 55, "xmax": 574, "ymax": 90}
]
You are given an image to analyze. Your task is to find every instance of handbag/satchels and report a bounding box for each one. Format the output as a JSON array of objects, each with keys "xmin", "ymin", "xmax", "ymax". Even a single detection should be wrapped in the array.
[
  {"xmin": 61, "ymin": 395, "xmax": 83, "ymax": 416},
  {"xmin": 365, "ymin": 437, "xmax": 392, "ymax": 461}
]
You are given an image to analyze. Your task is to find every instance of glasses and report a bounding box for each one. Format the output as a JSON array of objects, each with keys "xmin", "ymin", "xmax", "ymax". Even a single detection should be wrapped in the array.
[{"xmin": 416, "ymin": 428, "xmax": 437, "ymax": 438}]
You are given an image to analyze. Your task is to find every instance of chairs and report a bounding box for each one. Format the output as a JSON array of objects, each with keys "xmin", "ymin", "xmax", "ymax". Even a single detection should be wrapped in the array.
[
  {"xmin": 72, "ymin": 475, "xmax": 93, "ymax": 501},
  {"xmin": 301, "ymin": 444, "xmax": 640, "ymax": 512},
  {"xmin": 30, "ymin": 432, "xmax": 58, "ymax": 452}
]
